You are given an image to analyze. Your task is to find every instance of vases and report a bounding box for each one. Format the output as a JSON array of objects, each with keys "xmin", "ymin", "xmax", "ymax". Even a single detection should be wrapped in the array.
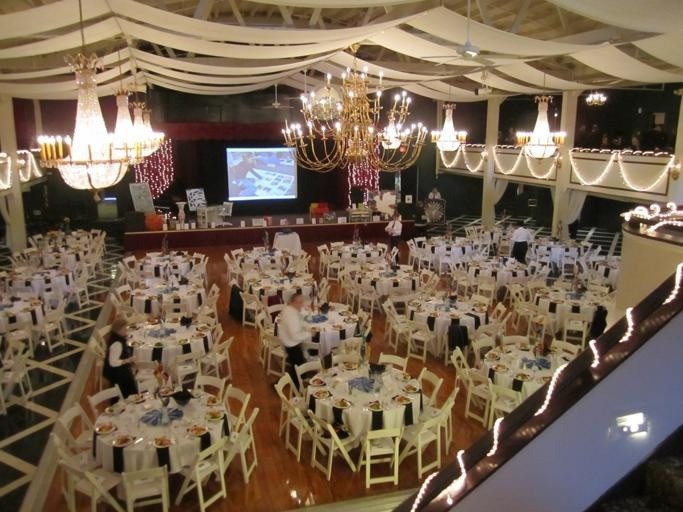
[{"xmin": 175, "ymin": 202, "xmax": 186, "ymax": 229}]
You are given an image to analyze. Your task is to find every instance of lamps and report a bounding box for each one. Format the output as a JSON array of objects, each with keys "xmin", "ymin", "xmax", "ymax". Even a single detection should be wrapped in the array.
[
  {"xmin": 128, "ymin": 58, "xmax": 154, "ymax": 158},
  {"xmin": 511, "ymin": 58, "xmax": 570, "ymax": 160},
  {"xmin": 583, "ymin": 86, "xmax": 608, "ymax": 107},
  {"xmin": 424, "ymin": 79, "xmax": 468, "ymax": 152},
  {"xmin": 35, "ymin": 0, "xmax": 132, "ymax": 191},
  {"xmin": 103, "ymin": 41, "xmax": 142, "ymax": 166},
  {"xmin": 279, "ymin": 41, "xmax": 426, "ymax": 180},
  {"xmin": 143, "ymin": 81, "xmax": 165, "ymax": 148}
]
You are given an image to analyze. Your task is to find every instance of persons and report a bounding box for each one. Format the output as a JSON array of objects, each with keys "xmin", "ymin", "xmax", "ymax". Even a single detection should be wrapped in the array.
[
  {"xmin": 507, "ymin": 218, "xmax": 536, "ymax": 267},
  {"xmin": 382, "ymin": 212, "xmax": 404, "ymax": 263},
  {"xmin": 274, "ymin": 286, "xmax": 323, "ymax": 392},
  {"xmin": 100, "ymin": 317, "xmax": 139, "ymax": 406},
  {"xmin": 427, "ymin": 187, "xmax": 443, "ymax": 201}
]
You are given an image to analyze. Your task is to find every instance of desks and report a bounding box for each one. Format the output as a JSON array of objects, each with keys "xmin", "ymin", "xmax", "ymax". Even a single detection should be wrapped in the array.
[{"xmin": 196, "ymin": 205, "xmax": 221, "ymax": 229}]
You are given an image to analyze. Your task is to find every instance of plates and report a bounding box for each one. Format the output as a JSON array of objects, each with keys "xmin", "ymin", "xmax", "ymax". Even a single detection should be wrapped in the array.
[
  {"xmin": 94, "ymin": 426, "xmax": 116, "ymax": 435},
  {"xmin": 103, "ymin": 385, "xmax": 224, "ymax": 421},
  {"xmin": 308, "ymin": 361, "xmax": 418, "ymax": 412},
  {"xmin": 111, "ymin": 437, "xmax": 133, "ymax": 447},
  {"xmin": 152, "ymin": 438, "xmax": 171, "ymax": 448},
  {"xmin": 408, "ymin": 294, "xmax": 487, "ymax": 320},
  {"xmin": 330, "ymin": 310, "xmax": 355, "ymax": 331},
  {"xmin": 485, "ymin": 342, "xmax": 558, "ymax": 381},
  {"xmin": 186, "ymin": 424, "xmax": 208, "ymax": 435},
  {"xmin": 127, "ymin": 312, "xmax": 211, "ymax": 348}
]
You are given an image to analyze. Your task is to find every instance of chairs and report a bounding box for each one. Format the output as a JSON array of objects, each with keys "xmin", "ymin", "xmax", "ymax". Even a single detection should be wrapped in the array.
[{"xmin": 216, "ymin": 201, "xmax": 233, "ymax": 227}]
